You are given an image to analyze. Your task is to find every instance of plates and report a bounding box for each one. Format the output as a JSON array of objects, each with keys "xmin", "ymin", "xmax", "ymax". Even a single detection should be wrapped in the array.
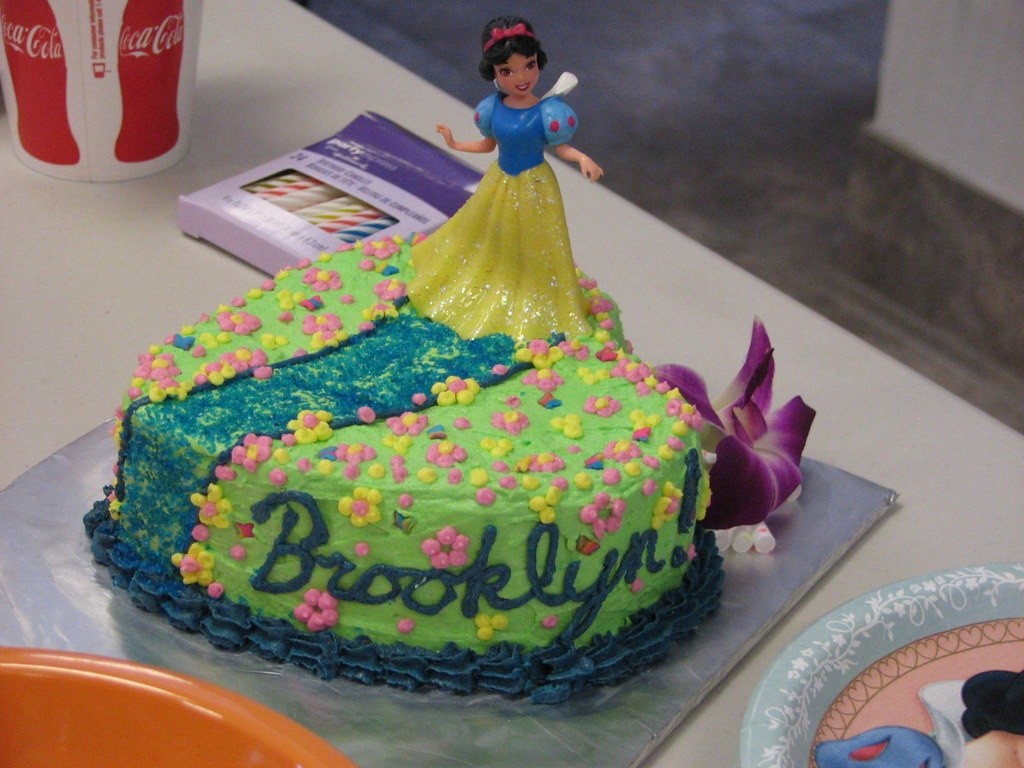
[{"xmin": 740, "ymin": 562, "xmax": 1024, "ymax": 768}]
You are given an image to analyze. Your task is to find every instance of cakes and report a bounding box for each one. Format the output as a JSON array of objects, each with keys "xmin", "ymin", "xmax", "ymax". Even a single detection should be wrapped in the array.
[{"xmin": 82, "ymin": 20, "xmax": 726, "ymax": 705}]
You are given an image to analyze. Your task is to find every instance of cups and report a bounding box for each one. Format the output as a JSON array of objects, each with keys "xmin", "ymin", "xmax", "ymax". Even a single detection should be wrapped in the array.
[{"xmin": 0, "ymin": 0, "xmax": 202, "ymax": 181}]
